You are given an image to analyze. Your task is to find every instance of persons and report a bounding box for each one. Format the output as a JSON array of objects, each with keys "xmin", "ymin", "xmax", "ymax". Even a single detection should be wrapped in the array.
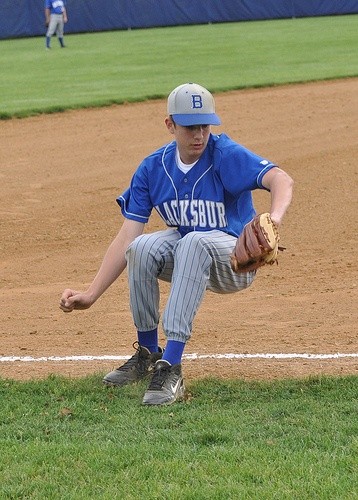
[
  {"xmin": 43, "ymin": 1, "xmax": 68, "ymax": 49},
  {"xmin": 59, "ymin": 80, "xmax": 295, "ymax": 406}
]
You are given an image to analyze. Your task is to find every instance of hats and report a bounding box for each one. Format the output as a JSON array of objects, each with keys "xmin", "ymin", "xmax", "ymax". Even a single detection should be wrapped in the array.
[{"xmin": 167, "ymin": 83, "xmax": 222, "ymax": 126}]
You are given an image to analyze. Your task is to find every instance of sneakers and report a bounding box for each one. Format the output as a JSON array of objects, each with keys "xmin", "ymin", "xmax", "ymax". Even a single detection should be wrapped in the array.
[
  {"xmin": 102, "ymin": 341, "xmax": 165, "ymax": 386},
  {"xmin": 142, "ymin": 359, "xmax": 186, "ymax": 406}
]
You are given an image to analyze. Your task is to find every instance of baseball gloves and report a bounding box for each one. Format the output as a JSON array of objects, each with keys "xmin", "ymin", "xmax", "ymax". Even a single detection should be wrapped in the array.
[{"xmin": 230, "ymin": 213, "xmax": 286, "ymax": 274}]
